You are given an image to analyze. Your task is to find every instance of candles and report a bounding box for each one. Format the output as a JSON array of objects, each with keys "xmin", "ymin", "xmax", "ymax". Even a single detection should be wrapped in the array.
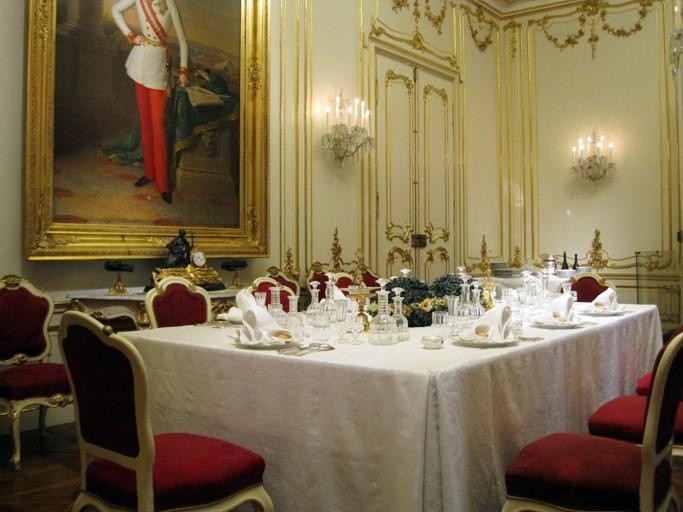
[
  {"xmin": 322, "ymin": 93, "xmax": 375, "ymax": 139},
  {"xmin": 570, "ymin": 135, "xmax": 614, "ymax": 169}
]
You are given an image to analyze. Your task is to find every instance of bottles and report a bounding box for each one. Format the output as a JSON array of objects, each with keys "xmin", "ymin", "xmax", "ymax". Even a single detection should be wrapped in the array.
[
  {"xmin": 400, "ymin": 269, "xmax": 410, "ymax": 277},
  {"xmin": 249, "ymin": 272, "xmax": 341, "ymax": 343},
  {"xmin": 454, "ymin": 266, "xmax": 573, "ymax": 336},
  {"xmin": 561, "ymin": 251, "xmax": 569, "ymax": 270},
  {"xmin": 573, "ymin": 253, "xmax": 580, "ymax": 269}
]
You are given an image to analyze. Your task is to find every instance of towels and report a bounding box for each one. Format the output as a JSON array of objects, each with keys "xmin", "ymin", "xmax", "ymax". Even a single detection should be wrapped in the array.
[
  {"xmin": 535, "ymin": 291, "xmax": 573, "ymax": 324},
  {"xmin": 458, "ymin": 301, "xmax": 515, "ymax": 341},
  {"xmin": 592, "ymin": 286, "xmax": 616, "ymax": 311}
]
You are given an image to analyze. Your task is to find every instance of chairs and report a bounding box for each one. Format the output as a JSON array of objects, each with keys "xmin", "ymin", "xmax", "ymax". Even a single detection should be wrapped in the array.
[
  {"xmin": 0, "ymin": 272, "xmax": 73, "ymax": 464},
  {"xmin": 145, "ymin": 276, "xmax": 214, "ymax": 327},
  {"xmin": 56, "ymin": 309, "xmax": 278, "ymax": 511},
  {"xmin": 500, "ymin": 330, "xmax": 683, "ymax": 512},
  {"xmin": 562, "ymin": 271, "xmax": 616, "ymax": 303},
  {"xmin": 238, "ymin": 263, "xmax": 384, "ymax": 331}
]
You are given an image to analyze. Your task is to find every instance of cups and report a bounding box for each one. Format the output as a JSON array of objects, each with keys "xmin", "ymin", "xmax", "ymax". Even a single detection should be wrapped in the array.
[
  {"xmin": 334, "ymin": 298, "xmax": 349, "ymax": 321},
  {"xmin": 444, "ymin": 295, "xmax": 455, "ymax": 314},
  {"xmin": 352, "ymin": 315, "xmax": 364, "ymax": 332},
  {"xmin": 430, "ymin": 311, "xmax": 450, "ymax": 339}
]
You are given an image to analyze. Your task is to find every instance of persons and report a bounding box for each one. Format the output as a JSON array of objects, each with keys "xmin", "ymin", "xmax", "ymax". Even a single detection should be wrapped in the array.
[{"xmin": 111, "ymin": 1, "xmax": 191, "ymax": 204}]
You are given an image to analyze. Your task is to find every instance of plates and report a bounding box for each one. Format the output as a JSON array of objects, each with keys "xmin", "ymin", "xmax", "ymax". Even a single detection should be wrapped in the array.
[
  {"xmin": 579, "ymin": 304, "xmax": 626, "ymax": 316},
  {"xmin": 530, "ymin": 315, "xmax": 583, "ymax": 329},
  {"xmin": 233, "ymin": 336, "xmax": 296, "ymax": 350},
  {"xmin": 452, "ymin": 337, "xmax": 517, "ymax": 347}
]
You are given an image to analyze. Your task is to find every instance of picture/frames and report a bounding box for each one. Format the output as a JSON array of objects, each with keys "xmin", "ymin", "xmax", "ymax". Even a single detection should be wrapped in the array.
[{"xmin": 21, "ymin": 1, "xmax": 272, "ymax": 263}]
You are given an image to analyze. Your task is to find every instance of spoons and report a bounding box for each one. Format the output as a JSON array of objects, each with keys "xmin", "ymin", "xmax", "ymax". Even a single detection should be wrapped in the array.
[{"xmin": 277, "ymin": 344, "xmax": 335, "ymax": 357}]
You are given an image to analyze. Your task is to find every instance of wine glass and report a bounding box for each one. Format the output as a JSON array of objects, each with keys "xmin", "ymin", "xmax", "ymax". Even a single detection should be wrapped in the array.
[{"xmin": 365, "ymin": 277, "xmax": 410, "ymax": 347}]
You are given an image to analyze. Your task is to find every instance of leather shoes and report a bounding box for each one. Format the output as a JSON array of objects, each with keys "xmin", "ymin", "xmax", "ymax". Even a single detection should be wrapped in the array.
[
  {"xmin": 161, "ymin": 192, "xmax": 171, "ymax": 204},
  {"xmin": 135, "ymin": 176, "xmax": 148, "ymax": 186}
]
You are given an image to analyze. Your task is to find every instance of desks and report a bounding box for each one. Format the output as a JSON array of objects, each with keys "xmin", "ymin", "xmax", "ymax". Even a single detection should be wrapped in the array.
[{"xmin": 67, "ymin": 282, "xmax": 251, "ymax": 329}]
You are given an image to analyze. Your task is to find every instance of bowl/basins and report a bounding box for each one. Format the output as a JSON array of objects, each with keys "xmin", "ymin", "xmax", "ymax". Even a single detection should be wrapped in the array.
[
  {"xmin": 577, "ymin": 267, "xmax": 591, "ymax": 272},
  {"xmin": 557, "ymin": 270, "xmax": 576, "ymax": 278},
  {"xmin": 422, "ymin": 336, "xmax": 443, "ymax": 349}
]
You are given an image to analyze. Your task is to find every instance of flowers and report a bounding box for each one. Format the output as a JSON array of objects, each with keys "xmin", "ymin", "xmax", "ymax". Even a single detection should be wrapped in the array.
[{"xmin": 366, "ymin": 273, "xmax": 446, "ymax": 316}]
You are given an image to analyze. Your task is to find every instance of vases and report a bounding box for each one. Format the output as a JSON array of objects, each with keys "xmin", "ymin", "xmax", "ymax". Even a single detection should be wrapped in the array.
[{"xmin": 404, "ymin": 316, "xmax": 431, "ymax": 327}]
